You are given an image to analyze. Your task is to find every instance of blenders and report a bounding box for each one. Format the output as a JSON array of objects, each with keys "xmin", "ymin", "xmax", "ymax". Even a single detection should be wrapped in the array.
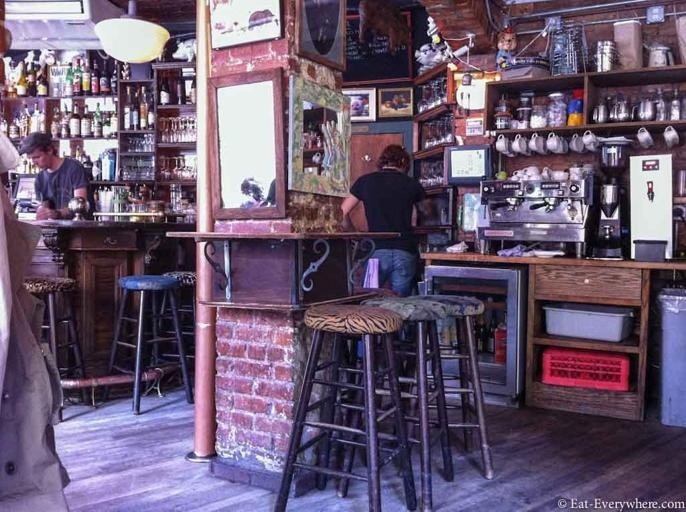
[{"xmin": 591, "ymin": 145, "xmax": 630, "ymax": 259}]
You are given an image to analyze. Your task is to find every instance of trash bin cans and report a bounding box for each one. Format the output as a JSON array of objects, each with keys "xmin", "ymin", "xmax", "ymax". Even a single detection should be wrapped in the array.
[{"xmin": 657, "ymin": 288, "xmax": 685, "ymax": 427}]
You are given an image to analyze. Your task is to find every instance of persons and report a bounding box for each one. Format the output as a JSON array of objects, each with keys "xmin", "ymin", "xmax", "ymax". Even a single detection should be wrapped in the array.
[
  {"xmin": 238, "ymin": 177, "xmax": 264, "ymax": 209},
  {"xmin": 17, "ymin": 131, "xmax": 95, "ymax": 219},
  {"xmin": 343, "ymin": 144, "xmax": 435, "ymax": 364},
  {"xmin": 0, "ymin": 125, "xmax": 73, "ymax": 511}
]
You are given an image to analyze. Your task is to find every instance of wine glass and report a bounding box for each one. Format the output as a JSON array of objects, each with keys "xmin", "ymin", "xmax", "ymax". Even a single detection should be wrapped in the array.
[
  {"xmin": 416, "ymin": 77, "xmax": 448, "ymax": 112},
  {"xmin": 159, "ymin": 117, "xmax": 196, "ymax": 142},
  {"xmin": 159, "ymin": 155, "xmax": 198, "ymax": 181},
  {"xmin": 424, "ymin": 115, "xmax": 455, "ymax": 150}
]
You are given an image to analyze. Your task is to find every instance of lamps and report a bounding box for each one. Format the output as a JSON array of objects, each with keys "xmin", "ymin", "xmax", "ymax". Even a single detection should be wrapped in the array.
[{"xmin": 94, "ymin": 0, "xmax": 170, "ymax": 64}]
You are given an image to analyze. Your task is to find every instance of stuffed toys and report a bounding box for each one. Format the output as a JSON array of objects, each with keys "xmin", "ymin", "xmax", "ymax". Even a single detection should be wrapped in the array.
[{"xmin": 496, "ymin": 28, "xmax": 517, "ymax": 68}]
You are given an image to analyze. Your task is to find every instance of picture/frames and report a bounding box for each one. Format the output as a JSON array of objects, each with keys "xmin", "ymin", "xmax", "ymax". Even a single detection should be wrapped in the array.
[
  {"xmin": 342, "ymin": 88, "xmax": 376, "ymax": 122},
  {"xmin": 295, "ymin": 0, "xmax": 347, "ymax": 72},
  {"xmin": 378, "ymin": 88, "xmax": 413, "ymax": 117},
  {"xmin": 209, "ymin": 0, "xmax": 285, "ymax": 51},
  {"xmin": 287, "ymin": 74, "xmax": 351, "ymax": 198}
]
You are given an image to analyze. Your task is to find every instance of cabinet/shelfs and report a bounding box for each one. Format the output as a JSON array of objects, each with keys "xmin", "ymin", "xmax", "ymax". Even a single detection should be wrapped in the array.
[
  {"xmin": 411, "ymin": 61, "xmax": 458, "ymax": 249},
  {"xmin": 524, "ymin": 263, "xmax": 649, "ymax": 421},
  {"xmin": 0, "ymin": 21, "xmax": 198, "ymax": 216},
  {"xmin": 483, "ymin": 64, "xmax": 686, "ymax": 204}
]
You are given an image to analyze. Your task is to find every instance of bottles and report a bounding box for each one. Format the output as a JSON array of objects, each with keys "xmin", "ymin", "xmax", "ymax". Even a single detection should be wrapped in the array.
[
  {"xmin": 124, "ymin": 85, "xmax": 154, "ymax": 131},
  {"xmin": 494, "ymin": 93, "xmax": 568, "ymax": 128},
  {"xmin": 476, "ymin": 310, "xmax": 507, "ymax": 363},
  {"xmin": 159, "ymin": 68, "xmax": 198, "ymax": 104},
  {"xmin": 75, "ymin": 146, "xmax": 117, "ymax": 182},
  {"xmin": 16, "ymin": 59, "xmax": 117, "ymax": 97},
  {"xmin": 170, "ymin": 184, "xmax": 182, "ymax": 212},
  {"xmin": 0, "ymin": 100, "xmax": 118, "ymax": 139},
  {"xmin": 657, "ymin": 85, "xmax": 686, "ymax": 122}
]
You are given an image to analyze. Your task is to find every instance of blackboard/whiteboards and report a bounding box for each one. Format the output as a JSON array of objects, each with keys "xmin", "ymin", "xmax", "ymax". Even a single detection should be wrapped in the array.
[{"xmin": 342, "ymin": 11, "xmax": 414, "ymax": 87}]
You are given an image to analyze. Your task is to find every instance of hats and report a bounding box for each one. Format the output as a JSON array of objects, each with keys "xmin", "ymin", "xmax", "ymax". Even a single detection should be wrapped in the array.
[{"xmin": 18, "ymin": 133, "xmax": 49, "ymax": 156}]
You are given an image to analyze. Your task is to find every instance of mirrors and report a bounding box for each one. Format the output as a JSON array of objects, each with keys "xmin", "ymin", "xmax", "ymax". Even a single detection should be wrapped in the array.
[{"xmin": 206, "ymin": 67, "xmax": 286, "ymax": 220}]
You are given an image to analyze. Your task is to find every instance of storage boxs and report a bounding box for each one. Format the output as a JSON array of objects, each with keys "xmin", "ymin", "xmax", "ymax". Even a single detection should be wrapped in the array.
[{"xmin": 542, "ymin": 305, "xmax": 634, "ymax": 343}]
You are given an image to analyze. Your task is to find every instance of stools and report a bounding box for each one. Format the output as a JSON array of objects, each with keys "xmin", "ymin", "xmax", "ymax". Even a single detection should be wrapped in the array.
[
  {"xmin": 22, "ymin": 276, "xmax": 92, "ymax": 422},
  {"xmin": 393, "ymin": 295, "xmax": 495, "ymax": 480},
  {"xmin": 275, "ymin": 304, "xmax": 417, "ymax": 512},
  {"xmin": 102, "ymin": 275, "xmax": 194, "ymax": 414},
  {"xmin": 338, "ymin": 296, "xmax": 454, "ymax": 512},
  {"xmin": 145, "ymin": 271, "xmax": 199, "ymax": 397}
]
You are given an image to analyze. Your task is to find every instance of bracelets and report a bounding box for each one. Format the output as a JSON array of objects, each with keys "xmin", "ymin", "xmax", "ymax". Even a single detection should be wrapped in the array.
[{"xmin": 54, "ymin": 209, "xmax": 61, "ymax": 219}]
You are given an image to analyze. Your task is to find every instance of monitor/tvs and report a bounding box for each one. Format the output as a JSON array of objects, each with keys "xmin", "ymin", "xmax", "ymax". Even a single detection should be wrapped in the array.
[{"xmin": 13, "ymin": 173, "xmax": 39, "ymax": 204}]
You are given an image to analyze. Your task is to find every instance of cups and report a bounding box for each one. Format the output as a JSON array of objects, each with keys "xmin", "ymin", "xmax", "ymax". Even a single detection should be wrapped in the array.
[
  {"xmin": 495, "ymin": 167, "xmax": 552, "ymax": 180},
  {"xmin": 597, "ymin": 41, "xmax": 616, "ymax": 71},
  {"xmin": 495, "ymin": 126, "xmax": 680, "ymax": 157},
  {"xmin": 676, "ymin": 170, "xmax": 686, "ymax": 197}
]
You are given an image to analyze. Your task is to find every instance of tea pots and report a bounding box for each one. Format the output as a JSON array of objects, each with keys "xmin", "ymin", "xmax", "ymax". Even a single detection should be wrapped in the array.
[
  {"xmin": 592, "ymin": 97, "xmax": 655, "ymax": 123},
  {"xmin": 643, "ymin": 42, "xmax": 675, "ymax": 68}
]
